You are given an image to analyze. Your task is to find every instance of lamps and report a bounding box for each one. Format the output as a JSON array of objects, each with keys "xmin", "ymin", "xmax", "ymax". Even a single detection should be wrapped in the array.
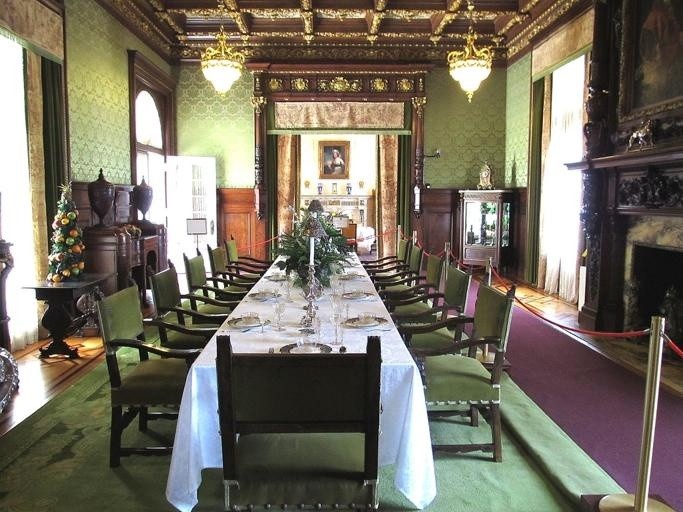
[
  {"xmin": 201, "ymin": 1, "xmax": 245, "ymax": 95},
  {"xmin": 447, "ymin": 2, "xmax": 497, "ymax": 104}
]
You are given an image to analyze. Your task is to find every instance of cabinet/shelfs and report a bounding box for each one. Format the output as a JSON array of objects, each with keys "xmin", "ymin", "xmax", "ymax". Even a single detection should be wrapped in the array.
[
  {"xmin": 456, "ymin": 189, "xmax": 521, "ymax": 284},
  {"xmin": 81, "ymin": 224, "xmax": 166, "ymax": 309}
]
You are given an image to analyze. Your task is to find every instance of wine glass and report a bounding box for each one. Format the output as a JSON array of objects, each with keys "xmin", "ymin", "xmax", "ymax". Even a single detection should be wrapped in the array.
[
  {"xmin": 272, "ymin": 265, "xmax": 293, "ymax": 332},
  {"xmin": 329, "ymin": 261, "xmax": 344, "ymax": 346}
]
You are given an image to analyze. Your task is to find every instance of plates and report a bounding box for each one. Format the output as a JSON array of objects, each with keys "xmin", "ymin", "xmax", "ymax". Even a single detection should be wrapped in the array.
[
  {"xmin": 342, "ymin": 292, "xmax": 374, "ymax": 300},
  {"xmin": 340, "ymin": 274, "xmax": 365, "ymax": 281},
  {"xmin": 345, "ymin": 316, "xmax": 388, "ymax": 329},
  {"xmin": 227, "ymin": 317, "xmax": 270, "ymax": 329},
  {"xmin": 264, "ymin": 275, "xmax": 291, "ymax": 281},
  {"xmin": 249, "ymin": 291, "xmax": 282, "ymax": 302},
  {"xmin": 281, "ymin": 343, "xmax": 332, "ymax": 354}
]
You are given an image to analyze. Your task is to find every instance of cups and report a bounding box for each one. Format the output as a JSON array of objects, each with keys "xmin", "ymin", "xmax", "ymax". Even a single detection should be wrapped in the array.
[
  {"xmin": 258, "ymin": 287, "xmax": 272, "ymax": 298},
  {"xmin": 352, "ymin": 288, "xmax": 365, "ymax": 297},
  {"xmin": 272, "ymin": 271, "xmax": 281, "ymax": 280},
  {"xmin": 297, "ymin": 328, "xmax": 319, "ymax": 352},
  {"xmin": 358, "ymin": 312, "xmax": 376, "ymax": 324},
  {"xmin": 347, "ymin": 270, "xmax": 358, "ymax": 279},
  {"xmin": 242, "ymin": 311, "xmax": 257, "ymax": 324}
]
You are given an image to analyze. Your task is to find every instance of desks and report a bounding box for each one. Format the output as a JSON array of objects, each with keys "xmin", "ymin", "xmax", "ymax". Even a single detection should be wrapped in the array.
[
  {"xmin": 18, "ymin": 272, "xmax": 114, "ymax": 359},
  {"xmin": 299, "ymin": 193, "xmax": 372, "ymax": 228}
]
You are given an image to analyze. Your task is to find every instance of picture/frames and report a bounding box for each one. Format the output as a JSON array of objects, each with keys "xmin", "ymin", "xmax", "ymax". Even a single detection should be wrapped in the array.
[
  {"xmin": 612, "ymin": 0, "xmax": 683, "ymax": 132},
  {"xmin": 316, "ymin": 141, "xmax": 350, "ymax": 180}
]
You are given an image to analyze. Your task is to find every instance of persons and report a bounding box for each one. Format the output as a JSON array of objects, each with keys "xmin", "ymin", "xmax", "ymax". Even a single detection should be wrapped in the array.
[{"xmin": 327, "ymin": 149, "xmax": 345, "ymax": 174}]
